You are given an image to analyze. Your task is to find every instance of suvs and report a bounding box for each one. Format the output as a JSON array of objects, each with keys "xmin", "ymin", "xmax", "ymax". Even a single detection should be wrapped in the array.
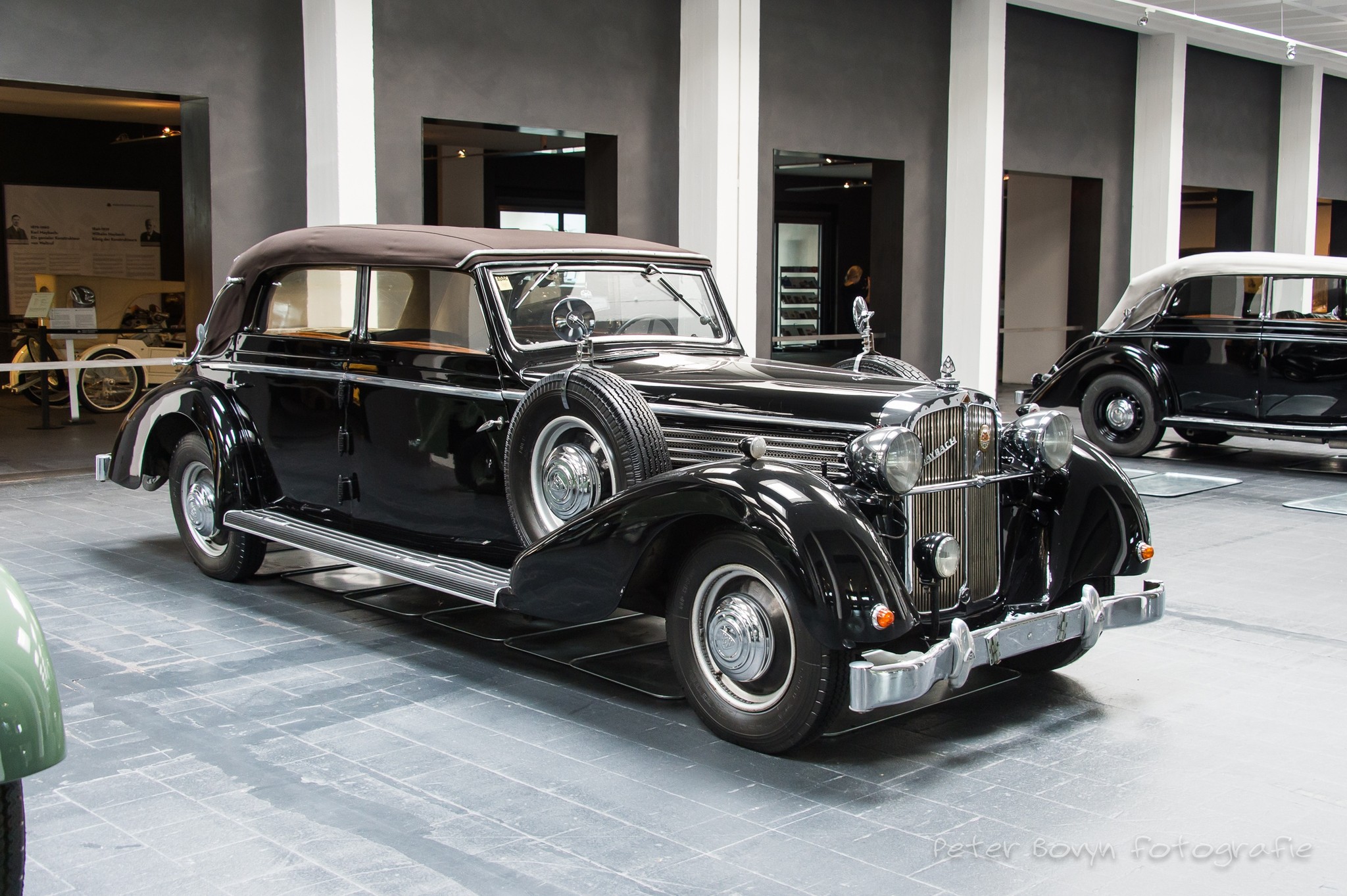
[
  {"xmin": 1017, "ymin": 251, "xmax": 1347, "ymax": 460},
  {"xmin": 108, "ymin": 224, "xmax": 1164, "ymax": 756}
]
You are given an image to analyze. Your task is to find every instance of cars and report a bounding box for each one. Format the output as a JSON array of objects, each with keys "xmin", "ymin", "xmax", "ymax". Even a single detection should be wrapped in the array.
[
  {"xmin": 0, "ymin": 570, "xmax": 69, "ymax": 896},
  {"xmin": 1, "ymin": 274, "xmax": 188, "ymax": 413}
]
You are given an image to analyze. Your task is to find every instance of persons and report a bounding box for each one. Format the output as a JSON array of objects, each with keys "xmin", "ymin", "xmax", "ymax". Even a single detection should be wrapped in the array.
[
  {"xmin": 140, "ymin": 218, "xmax": 161, "ymax": 243},
  {"xmin": 5, "ymin": 215, "xmax": 29, "ymax": 240}
]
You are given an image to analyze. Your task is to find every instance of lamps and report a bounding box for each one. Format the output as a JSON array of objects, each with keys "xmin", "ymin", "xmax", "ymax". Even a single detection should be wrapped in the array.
[{"xmin": 163, "ymin": 127, "xmax": 170, "ymax": 136}]
